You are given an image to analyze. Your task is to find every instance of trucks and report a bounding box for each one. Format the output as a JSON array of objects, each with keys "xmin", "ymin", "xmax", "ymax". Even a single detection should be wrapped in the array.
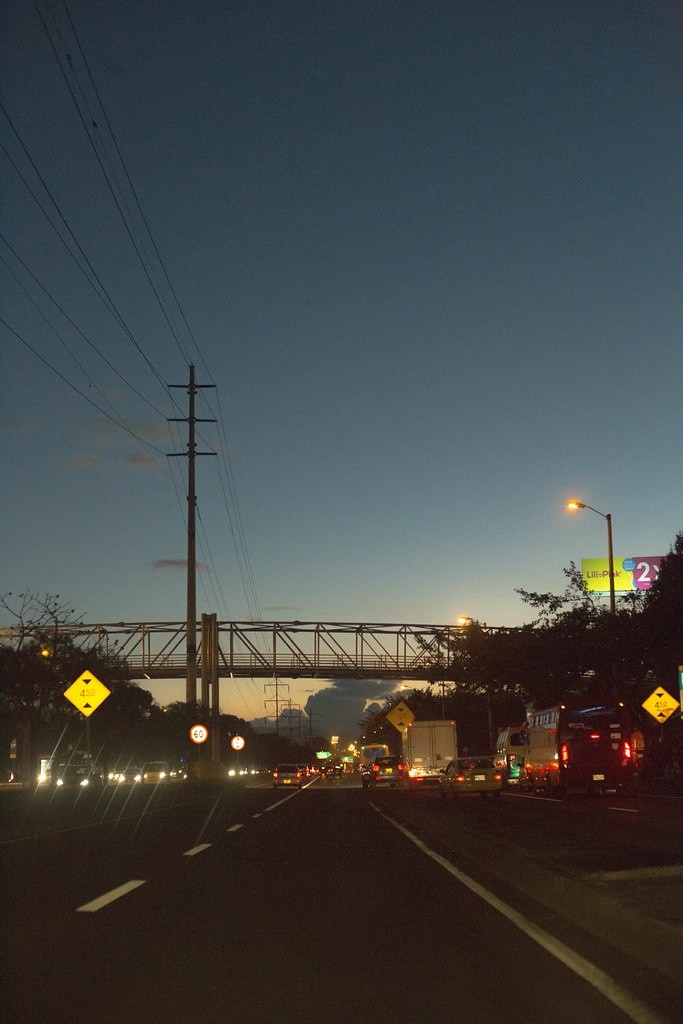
[{"xmin": 402, "ymin": 720, "xmax": 458, "ymax": 789}]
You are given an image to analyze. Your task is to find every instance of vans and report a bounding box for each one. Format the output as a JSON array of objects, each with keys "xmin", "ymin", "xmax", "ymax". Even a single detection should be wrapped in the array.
[{"xmin": 493, "ymin": 727, "xmax": 526, "ymax": 786}]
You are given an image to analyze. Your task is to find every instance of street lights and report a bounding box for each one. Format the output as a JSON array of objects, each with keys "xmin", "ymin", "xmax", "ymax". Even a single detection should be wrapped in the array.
[
  {"xmin": 459, "ymin": 615, "xmax": 495, "ymax": 752},
  {"xmin": 567, "ymin": 500, "xmax": 615, "ymax": 612}
]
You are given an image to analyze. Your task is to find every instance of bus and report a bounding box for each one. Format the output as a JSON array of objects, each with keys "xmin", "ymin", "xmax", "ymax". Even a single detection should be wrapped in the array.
[{"xmin": 526, "ymin": 703, "xmax": 634, "ymax": 800}]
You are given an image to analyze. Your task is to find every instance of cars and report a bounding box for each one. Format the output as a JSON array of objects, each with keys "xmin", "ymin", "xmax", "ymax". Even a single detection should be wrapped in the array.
[
  {"xmin": 517, "ymin": 757, "xmax": 530, "ymax": 793},
  {"xmin": 370, "ymin": 754, "xmax": 409, "ymax": 794},
  {"xmin": 272, "ymin": 764, "xmax": 302, "ymax": 790},
  {"xmin": 439, "ymin": 757, "xmax": 502, "ymax": 799},
  {"xmin": 295, "ymin": 744, "xmax": 390, "ymax": 788},
  {"xmin": 37, "ymin": 751, "xmax": 189, "ymax": 788}
]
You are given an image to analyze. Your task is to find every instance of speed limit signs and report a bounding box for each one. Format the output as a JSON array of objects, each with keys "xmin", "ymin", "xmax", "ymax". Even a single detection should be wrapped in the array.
[
  {"xmin": 231, "ymin": 736, "xmax": 245, "ymax": 751},
  {"xmin": 189, "ymin": 724, "xmax": 208, "ymax": 744}
]
